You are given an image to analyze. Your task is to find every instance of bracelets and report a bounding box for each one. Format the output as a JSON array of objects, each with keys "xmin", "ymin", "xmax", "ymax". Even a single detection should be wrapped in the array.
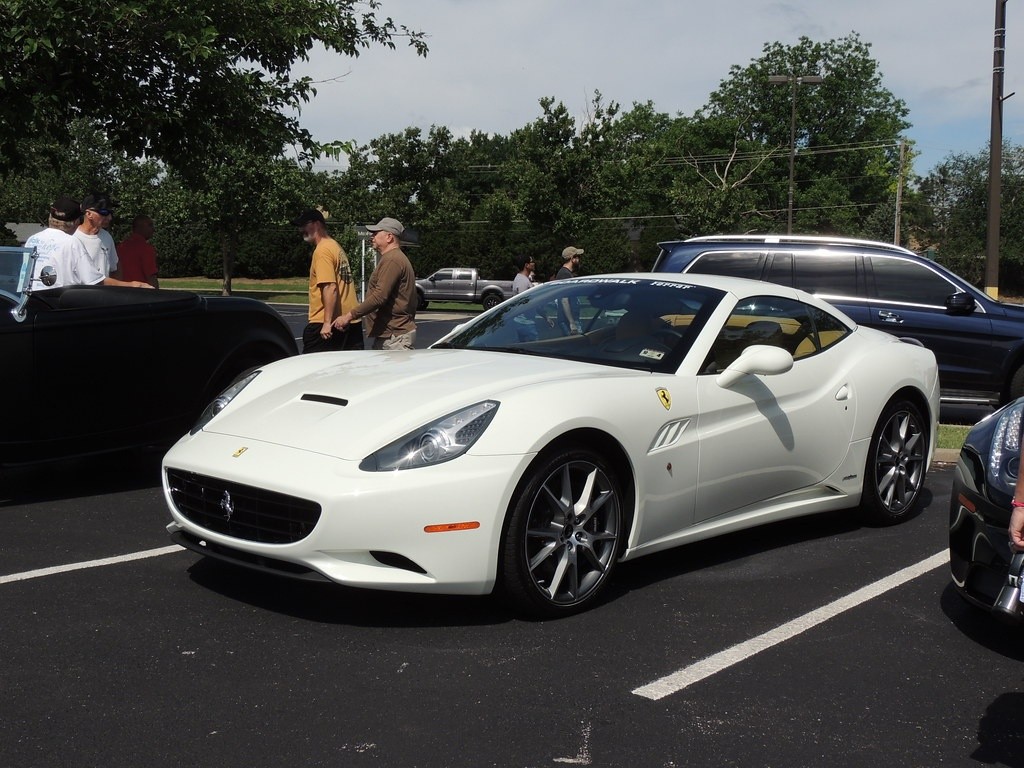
[{"xmin": 1011, "ymin": 496, "xmax": 1024, "ymax": 508}]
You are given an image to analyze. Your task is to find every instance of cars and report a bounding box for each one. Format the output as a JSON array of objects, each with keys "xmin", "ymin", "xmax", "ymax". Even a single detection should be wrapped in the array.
[
  {"xmin": 0, "ymin": 246, "xmax": 299, "ymax": 479},
  {"xmin": 947, "ymin": 398, "xmax": 1023, "ymax": 622}
]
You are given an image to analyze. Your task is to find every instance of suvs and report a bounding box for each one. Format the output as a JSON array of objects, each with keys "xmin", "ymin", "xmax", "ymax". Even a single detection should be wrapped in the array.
[{"xmin": 641, "ymin": 234, "xmax": 1024, "ymax": 406}]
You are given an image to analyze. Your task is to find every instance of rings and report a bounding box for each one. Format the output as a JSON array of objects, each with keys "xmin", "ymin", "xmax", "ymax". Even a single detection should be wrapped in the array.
[
  {"xmin": 1008, "ymin": 541, "xmax": 1011, "ymax": 547},
  {"xmin": 1011, "ymin": 542, "xmax": 1014, "ymax": 546}
]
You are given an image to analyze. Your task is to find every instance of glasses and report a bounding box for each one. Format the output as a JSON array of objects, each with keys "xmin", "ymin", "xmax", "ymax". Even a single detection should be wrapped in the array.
[
  {"xmin": 530, "ymin": 261, "xmax": 535, "ymax": 263},
  {"xmin": 90, "ymin": 208, "xmax": 114, "ymax": 216},
  {"xmin": 80, "ymin": 216, "xmax": 84, "ymax": 225},
  {"xmin": 575, "ymin": 255, "xmax": 580, "ymax": 258}
]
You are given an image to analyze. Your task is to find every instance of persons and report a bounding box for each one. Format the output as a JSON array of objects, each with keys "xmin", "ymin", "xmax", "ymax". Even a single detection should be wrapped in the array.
[
  {"xmin": 557, "ymin": 246, "xmax": 585, "ymax": 337},
  {"xmin": 17, "ymin": 195, "xmax": 159, "ymax": 293},
  {"xmin": 288, "ymin": 210, "xmax": 365, "ymax": 355},
  {"xmin": 511, "ymin": 256, "xmax": 554, "ymax": 345},
  {"xmin": 1007, "ymin": 434, "xmax": 1024, "ymax": 553},
  {"xmin": 329, "ymin": 217, "xmax": 417, "ymax": 350}
]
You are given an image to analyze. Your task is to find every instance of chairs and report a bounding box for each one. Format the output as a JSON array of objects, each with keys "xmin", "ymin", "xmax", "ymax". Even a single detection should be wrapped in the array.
[{"xmin": 738, "ymin": 321, "xmax": 785, "ymax": 349}]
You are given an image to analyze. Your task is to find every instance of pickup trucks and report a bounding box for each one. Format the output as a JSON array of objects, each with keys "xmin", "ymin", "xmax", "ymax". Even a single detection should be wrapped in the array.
[{"xmin": 414, "ymin": 268, "xmax": 513, "ymax": 311}]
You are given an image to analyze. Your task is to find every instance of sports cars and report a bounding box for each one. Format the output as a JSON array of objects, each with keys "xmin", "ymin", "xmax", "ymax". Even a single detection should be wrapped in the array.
[{"xmin": 160, "ymin": 269, "xmax": 941, "ymax": 621}]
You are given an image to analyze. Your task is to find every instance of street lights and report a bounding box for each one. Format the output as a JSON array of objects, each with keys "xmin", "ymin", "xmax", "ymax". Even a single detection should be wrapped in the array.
[{"xmin": 767, "ymin": 75, "xmax": 823, "ymax": 234}]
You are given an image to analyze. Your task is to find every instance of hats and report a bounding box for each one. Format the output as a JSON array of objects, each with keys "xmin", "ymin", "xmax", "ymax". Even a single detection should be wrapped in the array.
[
  {"xmin": 562, "ymin": 246, "xmax": 584, "ymax": 258},
  {"xmin": 51, "ymin": 199, "xmax": 83, "ymax": 222},
  {"xmin": 82, "ymin": 192, "xmax": 121, "ymax": 211},
  {"xmin": 365, "ymin": 218, "xmax": 405, "ymax": 238},
  {"xmin": 293, "ymin": 210, "xmax": 325, "ymax": 227}
]
